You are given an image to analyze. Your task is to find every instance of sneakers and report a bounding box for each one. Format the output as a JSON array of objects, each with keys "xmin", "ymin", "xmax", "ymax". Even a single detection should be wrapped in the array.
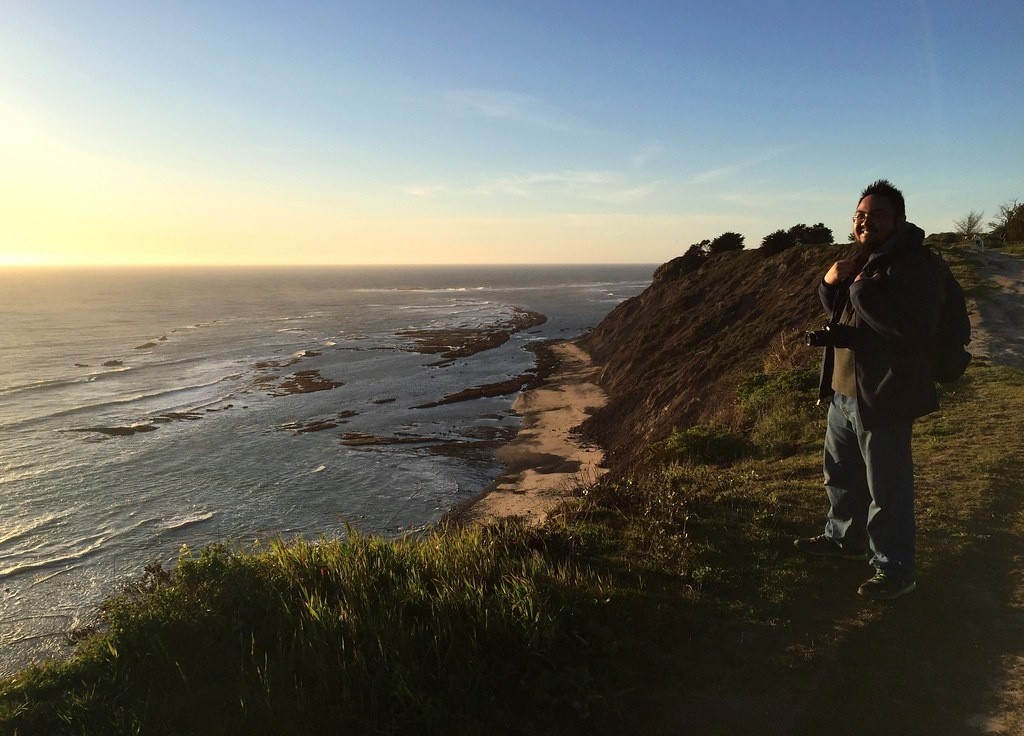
[
  {"xmin": 793, "ymin": 533, "xmax": 870, "ymax": 561},
  {"xmin": 857, "ymin": 572, "xmax": 918, "ymax": 601}
]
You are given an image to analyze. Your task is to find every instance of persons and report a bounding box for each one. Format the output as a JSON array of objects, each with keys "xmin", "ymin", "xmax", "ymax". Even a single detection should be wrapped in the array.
[{"xmin": 793, "ymin": 180, "xmax": 942, "ymax": 603}]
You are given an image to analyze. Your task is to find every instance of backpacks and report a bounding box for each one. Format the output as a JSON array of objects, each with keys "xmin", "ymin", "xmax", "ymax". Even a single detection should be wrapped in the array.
[{"xmin": 922, "ymin": 242, "xmax": 973, "ymax": 383}]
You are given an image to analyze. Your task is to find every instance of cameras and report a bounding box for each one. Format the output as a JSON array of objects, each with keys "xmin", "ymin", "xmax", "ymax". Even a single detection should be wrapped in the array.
[{"xmin": 804, "ymin": 322, "xmax": 852, "ymax": 347}]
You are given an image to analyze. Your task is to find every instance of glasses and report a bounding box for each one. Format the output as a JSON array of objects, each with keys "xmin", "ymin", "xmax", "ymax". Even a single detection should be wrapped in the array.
[{"xmin": 852, "ymin": 212, "xmax": 891, "ymax": 224}]
